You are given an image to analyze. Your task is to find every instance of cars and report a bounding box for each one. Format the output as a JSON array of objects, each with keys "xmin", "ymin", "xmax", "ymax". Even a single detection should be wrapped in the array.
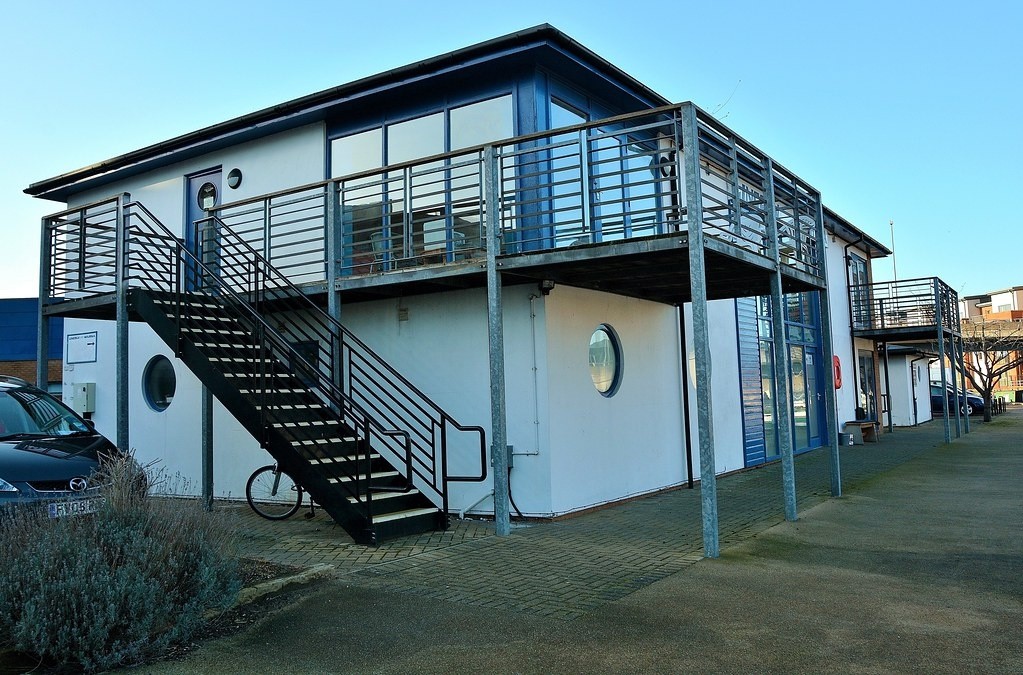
[{"xmin": 930, "ymin": 384, "xmax": 985, "ymax": 416}]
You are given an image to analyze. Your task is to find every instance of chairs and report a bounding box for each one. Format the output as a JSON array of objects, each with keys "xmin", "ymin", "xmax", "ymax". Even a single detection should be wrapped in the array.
[
  {"xmin": 762, "ymin": 214, "xmax": 816, "ymax": 274},
  {"xmin": 369, "ymin": 232, "xmax": 415, "ymax": 273},
  {"xmin": 719, "ymin": 213, "xmax": 763, "ymax": 253},
  {"xmin": 420, "ymin": 220, "xmax": 474, "ymax": 265}
]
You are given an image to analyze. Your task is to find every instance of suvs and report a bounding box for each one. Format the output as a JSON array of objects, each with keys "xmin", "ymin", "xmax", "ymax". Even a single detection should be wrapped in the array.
[
  {"xmin": 0, "ymin": 375, "xmax": 147, "ymax": 525},
  {"xmin": 930, "ymin": 379, "xmax": 977, "ymax": 396}
]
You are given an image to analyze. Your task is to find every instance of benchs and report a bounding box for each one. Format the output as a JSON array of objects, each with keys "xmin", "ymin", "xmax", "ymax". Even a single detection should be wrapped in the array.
[{"xmin": 845, "ymin": 422, "xmax": 878, "ymax": 445}]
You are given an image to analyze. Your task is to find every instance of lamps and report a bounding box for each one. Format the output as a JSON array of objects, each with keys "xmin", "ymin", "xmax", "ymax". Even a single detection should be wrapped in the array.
[
  {"xmin": 538, "ymin": 278, "xmax": 555, "ymax": 296},
  {"xmin": 226, "ymin": 168, "xmax": 242, "ymax": 190}
]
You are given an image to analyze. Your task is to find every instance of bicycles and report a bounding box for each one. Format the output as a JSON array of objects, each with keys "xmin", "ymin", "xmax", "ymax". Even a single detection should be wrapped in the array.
[{"xmin": 245, "ymin": 444, "xmax": 317, "ymax": 520}]
